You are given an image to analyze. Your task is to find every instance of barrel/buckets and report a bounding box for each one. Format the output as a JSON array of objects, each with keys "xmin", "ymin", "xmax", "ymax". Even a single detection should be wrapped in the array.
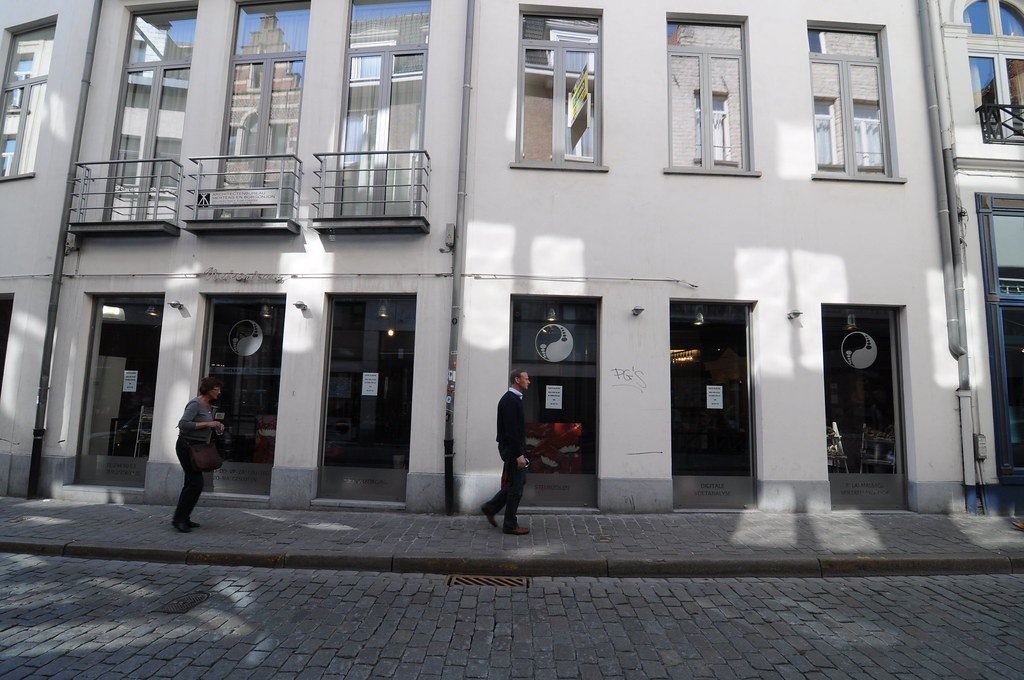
[{"xmin": 392, "ymin": 454, "xmax": 405, "ymax": 469}]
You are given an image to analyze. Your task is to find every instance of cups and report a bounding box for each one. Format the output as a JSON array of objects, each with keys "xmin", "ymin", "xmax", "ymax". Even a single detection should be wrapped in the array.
[{"xmin": 216, "ymin": 423, "xmax": 224, "ymax": 435}]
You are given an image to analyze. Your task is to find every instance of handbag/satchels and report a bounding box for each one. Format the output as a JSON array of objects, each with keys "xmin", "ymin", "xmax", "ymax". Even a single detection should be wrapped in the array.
[{"xmin": 190, "ymin": 442, "xmax": 223, "ymax": 472}]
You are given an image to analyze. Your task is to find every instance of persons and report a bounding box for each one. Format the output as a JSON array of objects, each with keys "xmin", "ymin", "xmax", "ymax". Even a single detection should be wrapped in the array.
[
  {"xmin": 171, "ymin": 378, "xmax": 224, "ymax": 532},
  {"xmin": 482, "ymin": 368, "xmax": 530, "ymax": 535}
]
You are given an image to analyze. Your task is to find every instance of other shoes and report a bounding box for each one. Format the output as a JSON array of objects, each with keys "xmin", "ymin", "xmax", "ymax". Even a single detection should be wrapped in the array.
[
  {"xmin": 188, "ymin": 521, "xmax": 200, "ymax": 528},
  {"xmin": 503, "ymin": 527, "xmax": 530, "ymax": 535},
  {"xmin": 481, "ymin": 504, "xmax": 498, "ymax": 527},
  {"xmin": 172, "ymin": 520, "xmax": 192, "ymax": 532}
]
